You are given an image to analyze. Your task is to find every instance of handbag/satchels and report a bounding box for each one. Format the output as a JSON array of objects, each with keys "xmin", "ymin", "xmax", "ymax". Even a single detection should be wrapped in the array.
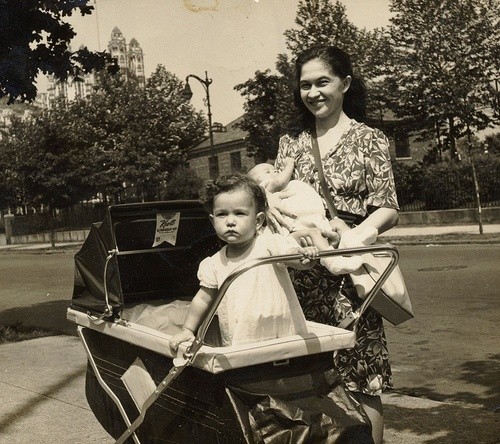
[{"xmin": 349, "ymin": 242, "xmax": 415, "ymax": 326}]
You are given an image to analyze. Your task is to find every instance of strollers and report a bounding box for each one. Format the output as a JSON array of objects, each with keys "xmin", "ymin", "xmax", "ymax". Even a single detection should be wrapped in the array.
[{"xmin": 67, "ymin": 198, "xmax": 400, "ymax": 444}]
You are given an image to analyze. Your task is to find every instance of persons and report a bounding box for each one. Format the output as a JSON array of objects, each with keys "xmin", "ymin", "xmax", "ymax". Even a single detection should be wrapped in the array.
[
  {"xmin": 168, "ymin": 174, "xmax": 319, "ymax": 352},
  {"xmin": 247, "ymin": 157, "xmax": 378, "ymax": 274},
  {"xmin": 265, "ymin": 45, "xmax": 400, "ymax": 444}
]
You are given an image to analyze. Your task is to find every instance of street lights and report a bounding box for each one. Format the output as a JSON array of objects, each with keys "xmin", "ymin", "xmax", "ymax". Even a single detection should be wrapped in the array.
[{"xmin": 181, "ymin": 70, "xmax": 220, "ymax": 184}]
